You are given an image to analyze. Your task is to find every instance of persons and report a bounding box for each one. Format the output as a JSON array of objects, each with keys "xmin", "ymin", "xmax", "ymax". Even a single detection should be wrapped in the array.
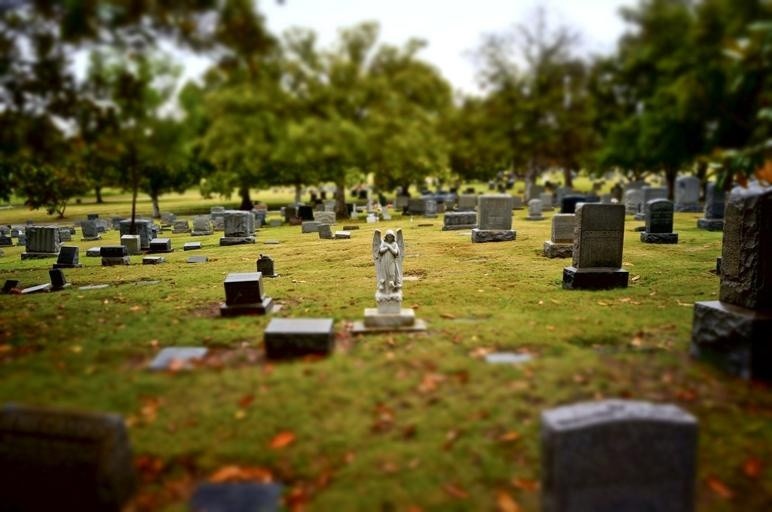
[{"xmin": 379, "ymin": 229, "xmax": 401, "ymax": 288}]
